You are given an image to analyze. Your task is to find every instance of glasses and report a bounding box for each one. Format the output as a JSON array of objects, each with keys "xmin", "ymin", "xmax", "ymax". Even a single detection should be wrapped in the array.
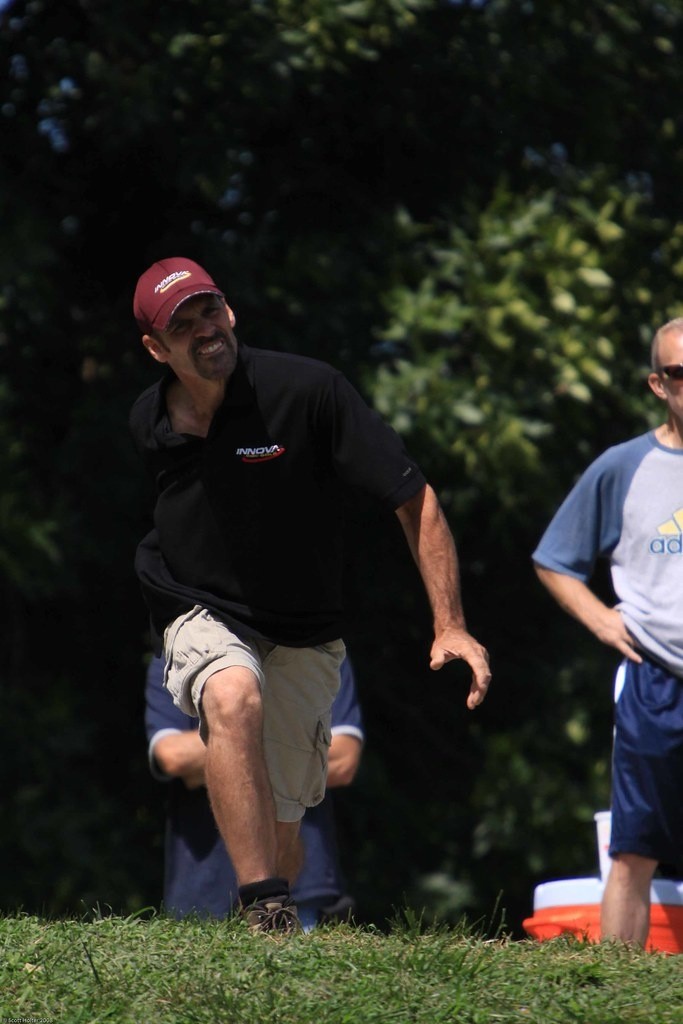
[{"xmin": 664, "ymin": 365, "xmax": 683, "ymax": 380}]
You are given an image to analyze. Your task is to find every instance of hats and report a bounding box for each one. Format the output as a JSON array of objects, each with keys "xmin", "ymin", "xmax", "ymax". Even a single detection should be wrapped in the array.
[{"xmin": 135, "ymin": 257, "xmax": 223, "ymax": 335}]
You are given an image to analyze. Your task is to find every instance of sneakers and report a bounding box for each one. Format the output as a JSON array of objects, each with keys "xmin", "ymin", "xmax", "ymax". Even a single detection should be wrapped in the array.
[{"xmin": 237, "ymin": 896, "xmax": 304, "ymax": 939}]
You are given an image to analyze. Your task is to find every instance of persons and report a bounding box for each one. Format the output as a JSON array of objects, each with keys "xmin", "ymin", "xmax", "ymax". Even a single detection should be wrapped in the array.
[
  {"xmin": 528, "ymin": 317, "xmax": 683, "ymax": 951},
  {"xmin": 120, "ymin": 257, "xmax": 493, "ymax": 927},
  {"xmin": 139, "ymin": 643, "xmax": 363, "ymax": 924}
]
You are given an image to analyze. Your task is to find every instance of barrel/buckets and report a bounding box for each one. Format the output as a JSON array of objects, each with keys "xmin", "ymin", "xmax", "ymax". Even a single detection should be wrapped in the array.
[{"xmin": 524, "ymin": 875, "xmax": 683, "ymax": 956}]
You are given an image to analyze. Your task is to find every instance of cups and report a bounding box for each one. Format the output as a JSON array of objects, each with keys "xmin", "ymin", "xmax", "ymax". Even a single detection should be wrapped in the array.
[{"xmin": 593, "ymin": 811, "xmax": 616, "ymax": 878}]
